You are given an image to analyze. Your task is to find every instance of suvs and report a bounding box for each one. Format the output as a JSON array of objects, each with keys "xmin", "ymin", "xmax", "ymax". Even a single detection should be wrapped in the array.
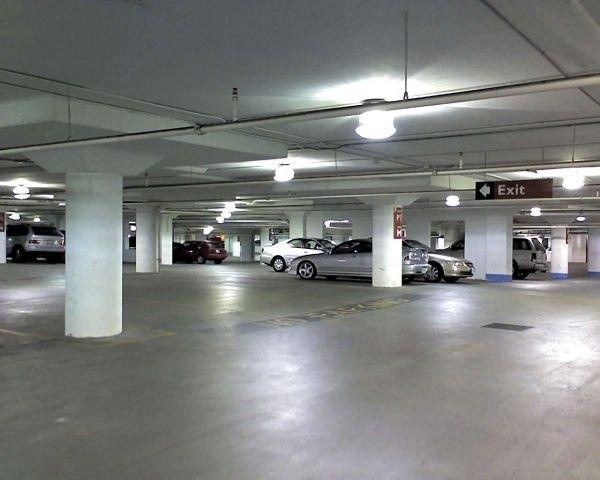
[
  {"xmin": 447, "ymin": 236, "xmax": 551, "ymax": 279},
  {"xmin": 7, "ymin": 220, "xmax": 66, "ymax": 264}
]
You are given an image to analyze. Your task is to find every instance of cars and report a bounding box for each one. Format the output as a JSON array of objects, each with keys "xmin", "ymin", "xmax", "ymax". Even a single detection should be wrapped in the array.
[
  {"xmin": 173, "ymin": 239, "xmax": 228, "ymax": 264},
  {"xmin": 402, "ymin": 239, "xmax": 475, "ymax": 282},
  {"xmin": 260, "ymin": 238, "xmax": 337, "ymax": 277},
  {"xmin": 289, "ymin": 238, "xmax": 429, "ymax": 287}
]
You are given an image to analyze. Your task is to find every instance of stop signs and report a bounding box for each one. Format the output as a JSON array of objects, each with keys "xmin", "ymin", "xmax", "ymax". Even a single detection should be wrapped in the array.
[{"xmin": 475, "ymin": 179, "xmax": 553, "ymax": 200}]
